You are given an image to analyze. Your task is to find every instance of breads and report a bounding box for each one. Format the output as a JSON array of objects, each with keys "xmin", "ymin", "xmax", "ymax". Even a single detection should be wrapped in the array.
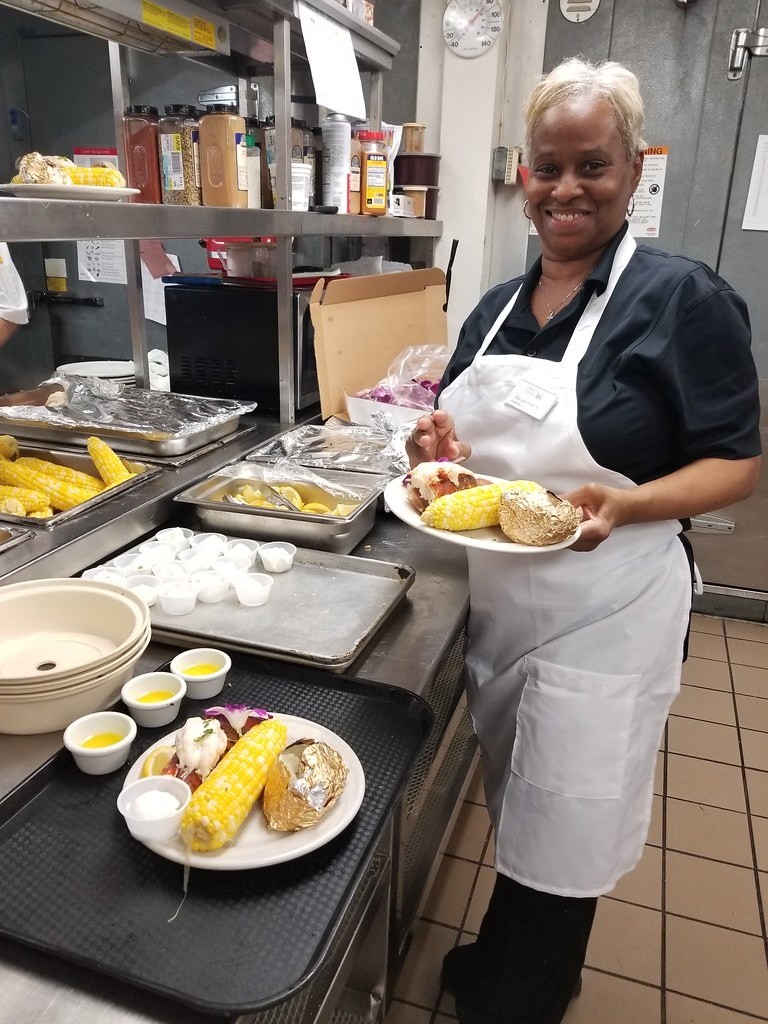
[{"xmin": 163, "ymin": 712, "xmax": 262, "ymax": 791}]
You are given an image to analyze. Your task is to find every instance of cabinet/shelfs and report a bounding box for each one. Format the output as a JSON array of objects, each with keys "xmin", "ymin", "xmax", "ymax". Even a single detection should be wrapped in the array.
[{"xmin": 0, "ymin": 1, "xmax": 442, "ymax": 580}]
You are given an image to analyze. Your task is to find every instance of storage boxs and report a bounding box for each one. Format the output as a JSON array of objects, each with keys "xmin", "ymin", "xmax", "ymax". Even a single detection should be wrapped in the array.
[{"xmin": 307, "ymin": 268, "xmax": 448, "ymax": 460}]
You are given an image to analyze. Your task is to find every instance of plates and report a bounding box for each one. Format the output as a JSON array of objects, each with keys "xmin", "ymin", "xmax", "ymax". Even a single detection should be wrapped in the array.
[
  {"xmin": 383, "ymin": 471, "xmax": 582, "ymax": 554},
  {"xmin": 0, "ymin": 183, "xmax": 142, "ymax": 202},
  {"xmin": 56, "ymin": 362, "xmax": 138, "ymax": 388},
  {"xmin": 114, "ymin": 707, "xmax": 366, "ymax": 871}
]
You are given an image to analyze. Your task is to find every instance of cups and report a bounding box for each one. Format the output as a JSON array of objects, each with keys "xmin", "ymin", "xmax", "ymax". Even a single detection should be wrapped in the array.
[{"xmin": 218, "ymin": 242, "xmax": 278, "ymax": 277}]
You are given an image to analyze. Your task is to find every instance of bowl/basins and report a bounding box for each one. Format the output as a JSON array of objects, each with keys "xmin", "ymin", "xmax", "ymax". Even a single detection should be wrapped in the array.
[
  {"xmin": 61, "ymin": 711, "xmax": 137, "ymax": 776},
  {"xmin": 0, "ymin": 577, "xmax": 152, "ymax": 735},
  {"xmin": 169, "ymin": 647, "xmax": 232, "ymax": 700},
  {"xmin": 82, "ymin": 528, "xmax": 298, "ymax": 615},
  {"xmin": 116, "ymin": 775, "xmax": 192, "ymax": 842},
  {"xmin": 120, "ymin": 672, "xmax": 186, "ymax": 728}
]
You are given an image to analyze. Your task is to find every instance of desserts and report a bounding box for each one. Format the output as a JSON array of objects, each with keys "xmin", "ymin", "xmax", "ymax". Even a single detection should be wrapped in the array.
[{"xmin": 405, "ymin": 461, "xmax": 493, "ymax": 513}]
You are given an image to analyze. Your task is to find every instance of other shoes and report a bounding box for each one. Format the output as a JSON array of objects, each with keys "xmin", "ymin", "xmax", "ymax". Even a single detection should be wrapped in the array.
[{"xmin": 442, "ymin": 943, "xmax": 586, "ymax": 1024}]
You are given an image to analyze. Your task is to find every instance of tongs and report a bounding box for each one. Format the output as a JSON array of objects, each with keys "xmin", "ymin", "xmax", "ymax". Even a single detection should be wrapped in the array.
[{"xmin": 222, "ymin": 483, "xmax": 301, "ymax": 512}]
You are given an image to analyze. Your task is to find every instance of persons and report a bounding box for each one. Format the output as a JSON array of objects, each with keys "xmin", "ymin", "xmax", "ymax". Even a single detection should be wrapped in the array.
[
  {"xmin": 402, "ymin": 50, "xmax": 765, "ymax": 1024},
  {"xmin": 0, "ymin": 241, "xmax": 33, "ymax": 354}
]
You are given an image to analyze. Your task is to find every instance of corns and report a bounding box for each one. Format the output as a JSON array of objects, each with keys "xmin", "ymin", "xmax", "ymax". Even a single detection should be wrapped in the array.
[
  {"xmin": 0, "ymin": 435, "xmax": 138, "ymax": 519},
  {"xmin": 181, "ymin": 717, "xmax": 287, "ymax": 850},
  {"xmin": 420, "ymin": 480, "xmax": 544, "ymax": 531}
]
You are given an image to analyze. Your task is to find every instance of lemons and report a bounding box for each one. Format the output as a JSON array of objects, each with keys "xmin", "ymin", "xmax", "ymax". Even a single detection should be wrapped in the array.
[
  {"xmin": 140, "ymin": 745, "xmax": 177, "ymax": 779},
  {"xmin": 232, "ymin": 485, "xmax": 359, "ymax": 516}
]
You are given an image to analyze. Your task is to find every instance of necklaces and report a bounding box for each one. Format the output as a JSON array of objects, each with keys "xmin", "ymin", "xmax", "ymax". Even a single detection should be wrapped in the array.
[{"xmin": 534, "ymin": 270, "xmax": 584, "ymax": 324}]
